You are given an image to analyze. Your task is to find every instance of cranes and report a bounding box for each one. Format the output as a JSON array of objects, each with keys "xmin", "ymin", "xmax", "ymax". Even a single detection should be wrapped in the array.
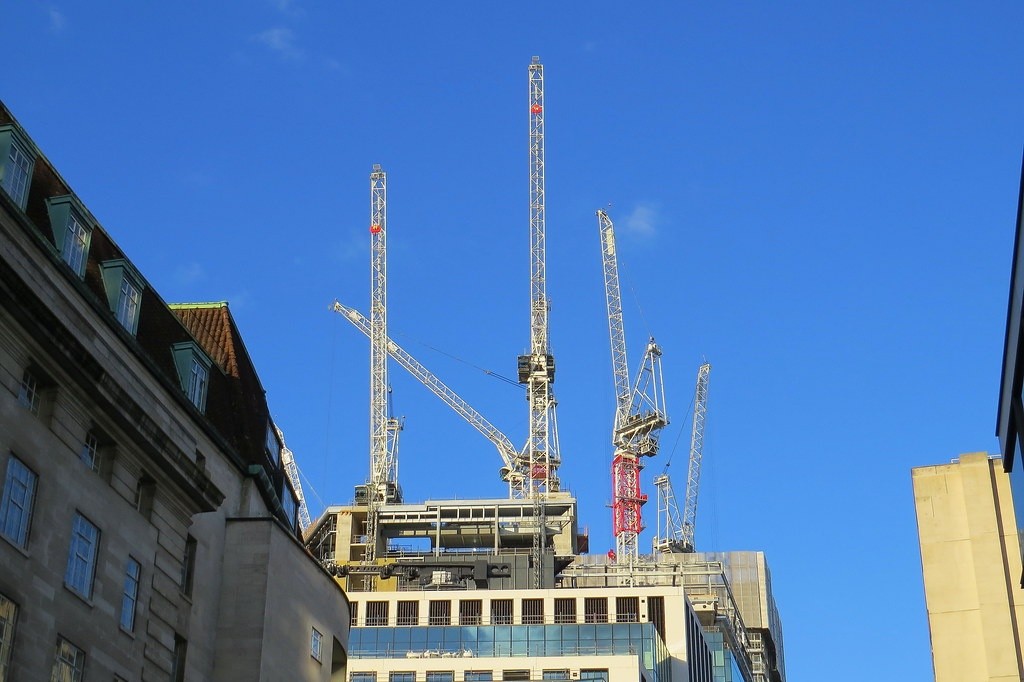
[
  {"xmin": 326, "ymin": 54, "xmax": 712, "ymax": 588},
  {"xmin": 271, "ymin": 418, "xmax": 325, "ymax": 536}
]
